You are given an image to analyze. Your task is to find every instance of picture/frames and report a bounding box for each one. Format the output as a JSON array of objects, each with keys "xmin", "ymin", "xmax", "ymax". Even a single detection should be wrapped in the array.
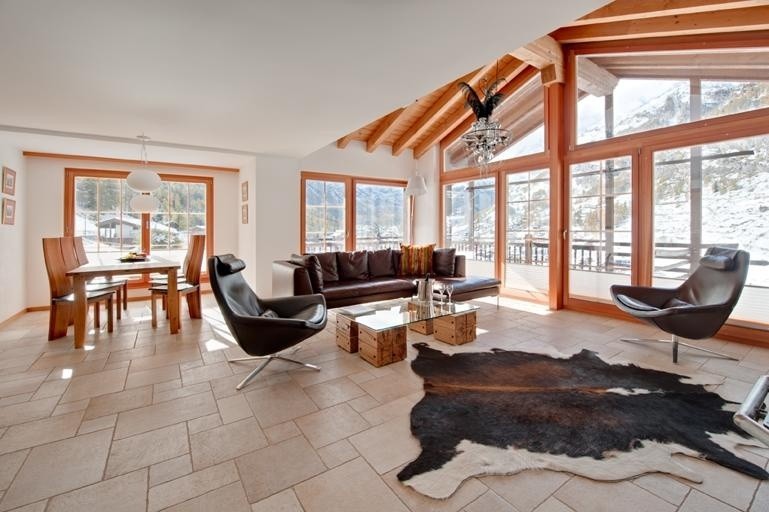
[
  {"xmin": 2, "ymin": 198, "xmax": 16, "ymax": 225},
  {"xmin": 241, "ymin": 181, "xmax": 249, "ymax": 202},
  {"xmin": 2, "ymin": 166, "xmax": 16, "ymax": 195},
  {"xmin": 242, "ymin": 204, "xmax": 248, "ymax": 225}
]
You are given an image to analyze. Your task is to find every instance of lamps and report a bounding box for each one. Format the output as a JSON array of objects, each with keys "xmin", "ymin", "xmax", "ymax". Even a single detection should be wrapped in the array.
[
  {"xmin": 130, "ymin": 194, "xmax": 161, "ymax": 214},
  {"xmin": 404, "ymin": 159, "xmax": 428, "ymax": 196},
  {"xmin": 456, "ymin": 58, "xmax": 514, "ymax": 175},
  {"xmin": 126, "ymin": 133, "xmax": 161, "ymax": 193}
]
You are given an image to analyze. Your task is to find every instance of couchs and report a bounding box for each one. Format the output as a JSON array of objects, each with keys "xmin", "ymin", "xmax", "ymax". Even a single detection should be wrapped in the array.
[{"xmin": 271, "ymin": 255, "xmax": 466, "ymax": 311}]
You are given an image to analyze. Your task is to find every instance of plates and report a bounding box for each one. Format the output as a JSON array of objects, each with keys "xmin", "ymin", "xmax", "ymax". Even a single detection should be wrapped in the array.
[{"xmin": 118, "ymin": 257, "xmax": 144, "ymax": 262}]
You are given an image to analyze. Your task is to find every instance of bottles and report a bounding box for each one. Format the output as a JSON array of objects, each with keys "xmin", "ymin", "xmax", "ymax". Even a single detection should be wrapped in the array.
[{"xmin": 425, "ymin": 273, "xmax": 430, "ymax": 281}]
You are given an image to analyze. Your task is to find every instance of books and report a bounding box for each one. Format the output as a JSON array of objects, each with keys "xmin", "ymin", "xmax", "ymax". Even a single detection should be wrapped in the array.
[{"xmin": 338, "ymin": 305, "xmax": 376, "ymax": 317}]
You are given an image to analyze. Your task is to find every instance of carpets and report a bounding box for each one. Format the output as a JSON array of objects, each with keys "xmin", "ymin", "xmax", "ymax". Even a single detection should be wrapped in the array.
[{"xmin": 397, "ymin": 342, "xmax": 769, "ymax": 500}]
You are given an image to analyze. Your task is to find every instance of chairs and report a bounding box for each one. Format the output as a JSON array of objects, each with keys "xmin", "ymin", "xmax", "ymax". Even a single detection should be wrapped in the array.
[
  {"xmin": 209, "ymin": 254, "xmax": 327, "ymax": 390},
  {"xmin": 610, "ymin": 247, "xmax": 749, "ymax": 364},
  {"xmin": 40, "ymin": 237, "xmax": 128, "ymax": 341},
  {"xmin": 148, "ymin": 234, "xmax": 205, "ymax": 331}
]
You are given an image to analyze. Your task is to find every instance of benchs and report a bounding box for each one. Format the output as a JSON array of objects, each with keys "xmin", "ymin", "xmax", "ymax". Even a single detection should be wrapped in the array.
[{"xmin": 433, "ymin": 277, "xmax": 500, "ymax": 313}]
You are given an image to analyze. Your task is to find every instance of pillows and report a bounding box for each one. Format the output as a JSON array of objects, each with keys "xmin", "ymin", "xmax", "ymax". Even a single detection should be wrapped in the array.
[{"xmin": 290, "ymin": 242, "xmax": 456, "ymax": 292}]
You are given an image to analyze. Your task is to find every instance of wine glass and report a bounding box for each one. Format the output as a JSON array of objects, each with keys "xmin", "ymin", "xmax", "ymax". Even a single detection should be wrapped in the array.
[
  {"xmin": 439, "ymin": 305, "xmax": 452, "ymax": 315},
  {"xmin": 437, "ymin": 283, "xmax": 453, "ymax": 305}
]
